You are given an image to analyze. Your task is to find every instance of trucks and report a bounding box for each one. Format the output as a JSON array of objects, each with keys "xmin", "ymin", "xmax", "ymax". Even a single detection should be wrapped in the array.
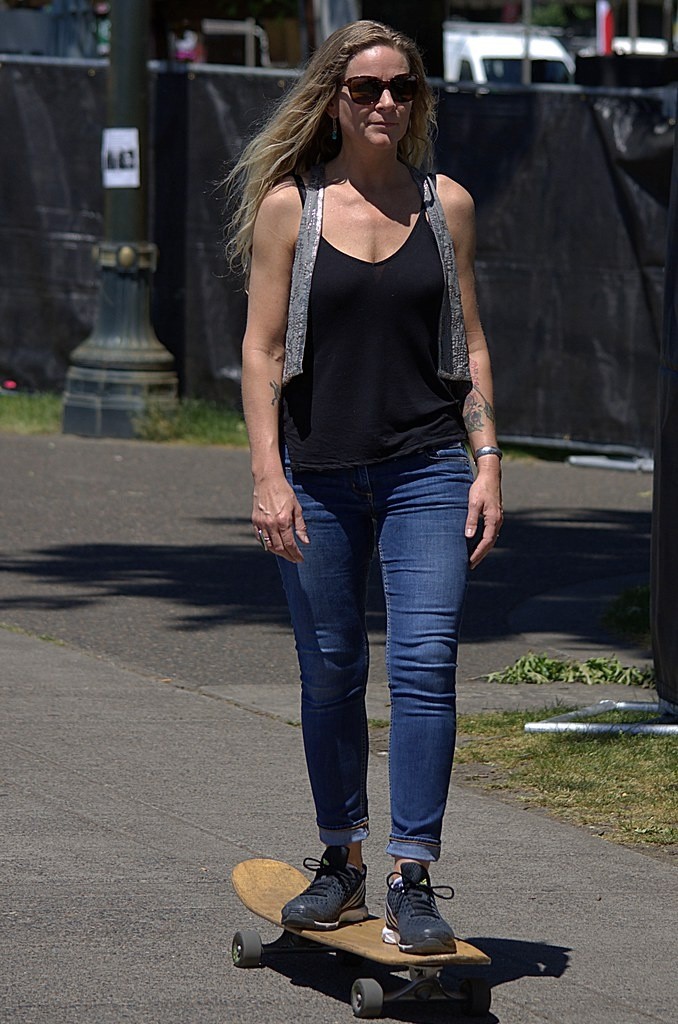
[{"xmin": 444, "ymin": 20, "xmax": 579, "ymax": 87}]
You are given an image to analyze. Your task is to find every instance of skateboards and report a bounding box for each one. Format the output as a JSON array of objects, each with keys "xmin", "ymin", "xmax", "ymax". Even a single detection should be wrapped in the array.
[{"xmin": 232, "ymin": 859, "xmax": 493, "ymax": 1017}]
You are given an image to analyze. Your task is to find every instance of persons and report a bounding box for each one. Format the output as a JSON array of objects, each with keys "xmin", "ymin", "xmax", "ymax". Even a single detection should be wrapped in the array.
[{"xmin": 219, "ymin": 19, "xmax": 508, "ymax": 956}]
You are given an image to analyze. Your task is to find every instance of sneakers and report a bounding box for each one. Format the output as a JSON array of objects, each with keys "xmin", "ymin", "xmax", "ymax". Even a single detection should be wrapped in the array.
[
  {"xmin": 280, "ymin": 844, "xmax": 368, "ymax": 931},
  {"xmin": 382, "ymin": 862, "xmax": 457, "ymax": 953}
]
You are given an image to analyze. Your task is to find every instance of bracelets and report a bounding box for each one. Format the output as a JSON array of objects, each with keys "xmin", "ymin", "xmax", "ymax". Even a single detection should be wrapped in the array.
[{"xmin": 472, "ymin": 445, "xmax": 502, "ymax": 466}]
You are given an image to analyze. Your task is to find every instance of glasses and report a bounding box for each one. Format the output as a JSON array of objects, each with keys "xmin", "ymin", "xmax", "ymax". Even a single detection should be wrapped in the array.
[{"xmin": 339, "ymin": 73, "xmax": 417, "ymax": 105}]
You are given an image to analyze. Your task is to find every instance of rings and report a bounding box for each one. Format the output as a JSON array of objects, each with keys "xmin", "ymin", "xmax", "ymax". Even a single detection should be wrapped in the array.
[
  {"xmin": 257, "ymin": 528, "xmax": 271, "ymax": 553},
  {"xmin": 495, "ymin": 533, "xmax": 500, "ymax": 538}
]
